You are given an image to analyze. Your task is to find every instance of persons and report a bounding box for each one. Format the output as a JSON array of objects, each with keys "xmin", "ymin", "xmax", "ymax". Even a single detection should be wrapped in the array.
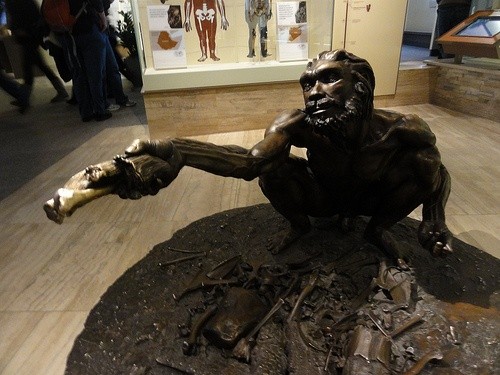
[
  {"xmin": 436, "ymin": 0, "xmax": 469, "ymax": 59},
  {"xmin": 3, "ymin": 0, "xmax": 68, "ymax": 105},
  {"xmin": 123, "ymin": 49, "xmax": 454, "ymax": 268},
  {"xmin": 0, "ymin": 68, "xmax": 31, "ymax": 112},
  {"xmin": 63, "ymin": 1, "xmax": 138, "ymax": 121}
]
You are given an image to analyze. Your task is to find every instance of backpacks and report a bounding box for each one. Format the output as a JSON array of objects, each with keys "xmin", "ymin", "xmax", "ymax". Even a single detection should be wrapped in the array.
[{"xmin": 41, "ymin": 0, "xmax": 92, "ymax": 33}]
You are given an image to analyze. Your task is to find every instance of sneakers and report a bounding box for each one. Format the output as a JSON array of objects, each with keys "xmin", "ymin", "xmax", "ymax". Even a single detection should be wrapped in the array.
[{"xmin": 106, "ymin": 104, "xmax": 120, "ymax": 111}]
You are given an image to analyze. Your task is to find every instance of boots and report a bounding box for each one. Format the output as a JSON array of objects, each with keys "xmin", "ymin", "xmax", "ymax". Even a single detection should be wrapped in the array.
[
  {"xmin": 10, "ymin": 84, "xmax": 31, "ymax": 106},
  {"xmin": 51, "ymin": 77, "xmax": 69, "ymax": 102}
]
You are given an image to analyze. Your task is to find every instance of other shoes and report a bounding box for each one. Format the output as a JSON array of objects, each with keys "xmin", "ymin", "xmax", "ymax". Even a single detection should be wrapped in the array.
[
  {"xmin": 125, "ymin": 98, "xmax": 137, "ymax": 107},
  {"xmin": 82, "ymin": 113, "xmax": 95, "ymax": 122},
  {"xmin": 96, "ymin": 112, "xmax": 112, "ymax": 121}
]
